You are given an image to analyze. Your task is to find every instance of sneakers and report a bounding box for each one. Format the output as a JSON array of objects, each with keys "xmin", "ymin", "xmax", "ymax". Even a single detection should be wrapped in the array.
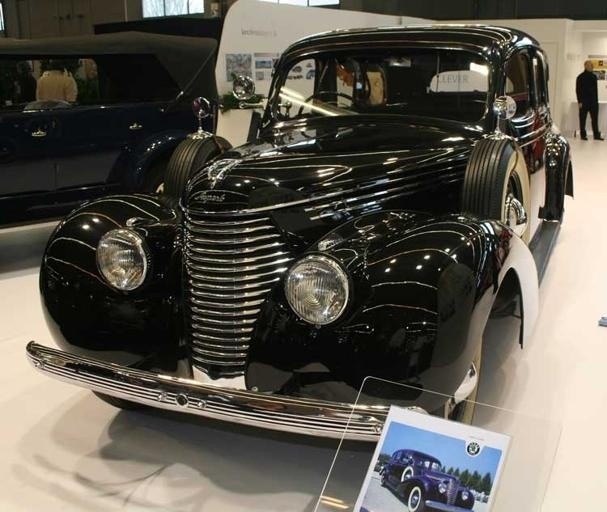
[{"xmin": 581, "ymin": 136, "xmax": 604, "ymax": 140}]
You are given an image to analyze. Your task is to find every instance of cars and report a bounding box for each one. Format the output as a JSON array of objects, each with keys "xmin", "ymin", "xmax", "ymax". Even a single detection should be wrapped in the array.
[
  {"xmin": 24, "ymin": 23, "xmax": 573, "ymax": 456},
  {"xmin": 379, "ymin": 450, "xmax": 474, "ymax": 512},
  {"xmin": 1, "ymin": 29, "xmax": 218, "ymax": 233}
]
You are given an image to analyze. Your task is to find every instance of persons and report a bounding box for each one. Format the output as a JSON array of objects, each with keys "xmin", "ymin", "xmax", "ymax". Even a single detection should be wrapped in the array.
[{"xmin": 573, "ymin": 59, "xmax": 603, "ymax": 141}]
